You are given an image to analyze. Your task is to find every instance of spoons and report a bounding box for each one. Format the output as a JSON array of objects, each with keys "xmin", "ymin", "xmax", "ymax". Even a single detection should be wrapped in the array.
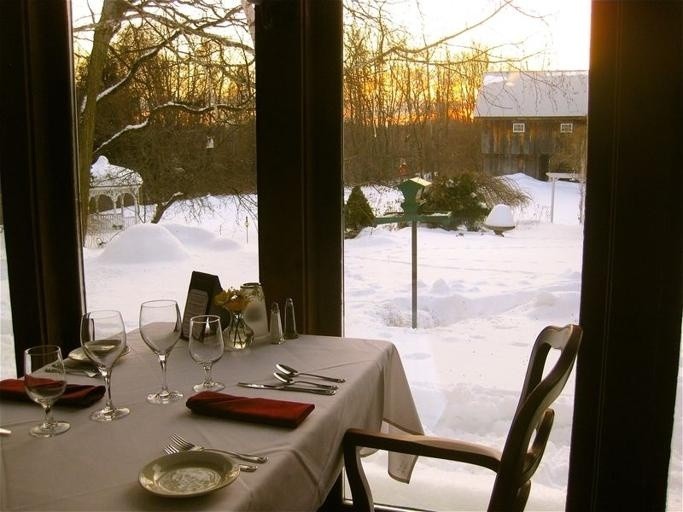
[{"xmin": 274, "ymin": 363, "xmax": 345, "ymax": 391}]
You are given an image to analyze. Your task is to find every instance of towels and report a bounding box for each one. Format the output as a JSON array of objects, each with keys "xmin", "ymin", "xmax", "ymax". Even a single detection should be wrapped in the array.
[
  {"xmin": 185, "ymin": 390, "xmax": 315, "ymax": 431},
  {"xmin": 0, "ymin": 375, "xmax": 106, "ymax": 409}
]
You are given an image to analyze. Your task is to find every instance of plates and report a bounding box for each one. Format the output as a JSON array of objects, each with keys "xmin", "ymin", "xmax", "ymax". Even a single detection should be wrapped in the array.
[{"xmin": 138, "ymin": 451, "xmax": 242, "ymax": 502}]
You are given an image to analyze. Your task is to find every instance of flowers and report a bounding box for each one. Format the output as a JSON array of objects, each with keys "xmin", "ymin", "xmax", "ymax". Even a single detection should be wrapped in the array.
[{"xmin": 213, "ymin": 286, "xmax": 255, "ymax": 349}]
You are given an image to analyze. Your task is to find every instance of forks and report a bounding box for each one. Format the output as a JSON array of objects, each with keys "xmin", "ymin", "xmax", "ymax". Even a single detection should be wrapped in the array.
[{"xmin": 163, "ymin": 434, "xmax": 267, "ymax": 472}]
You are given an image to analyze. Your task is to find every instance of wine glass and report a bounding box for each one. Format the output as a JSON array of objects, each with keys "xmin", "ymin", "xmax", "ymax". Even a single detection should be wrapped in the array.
[{"xmin": 25, "ymin": 299, "xmax": 225, "ymax": 440}]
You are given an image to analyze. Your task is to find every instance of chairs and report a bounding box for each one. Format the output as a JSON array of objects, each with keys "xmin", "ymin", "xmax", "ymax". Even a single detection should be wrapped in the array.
[{"xmin": 332, "ymin": 323, "xmax": 583, "ymax": 512}]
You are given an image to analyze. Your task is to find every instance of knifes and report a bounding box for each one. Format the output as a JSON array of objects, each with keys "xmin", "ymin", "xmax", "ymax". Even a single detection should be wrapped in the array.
[{"xmin": 237, "ymin": 382, "xmax": 336, "ymax": 397}]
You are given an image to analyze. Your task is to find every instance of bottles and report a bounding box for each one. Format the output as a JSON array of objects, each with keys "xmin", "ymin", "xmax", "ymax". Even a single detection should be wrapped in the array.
[
  {"xmin": 240, "ymin": 283, "xmax": 269, "ymax": 338},
  {"xmin": 268, "ymin": 299, "xmax": 298, "ymax": 344}
]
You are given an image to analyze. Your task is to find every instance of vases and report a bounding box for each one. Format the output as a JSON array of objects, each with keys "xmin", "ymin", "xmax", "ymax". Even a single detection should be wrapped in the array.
[{"xmin": 220, "ymin": 312, "xmax": 253, "ymax": 349}]
